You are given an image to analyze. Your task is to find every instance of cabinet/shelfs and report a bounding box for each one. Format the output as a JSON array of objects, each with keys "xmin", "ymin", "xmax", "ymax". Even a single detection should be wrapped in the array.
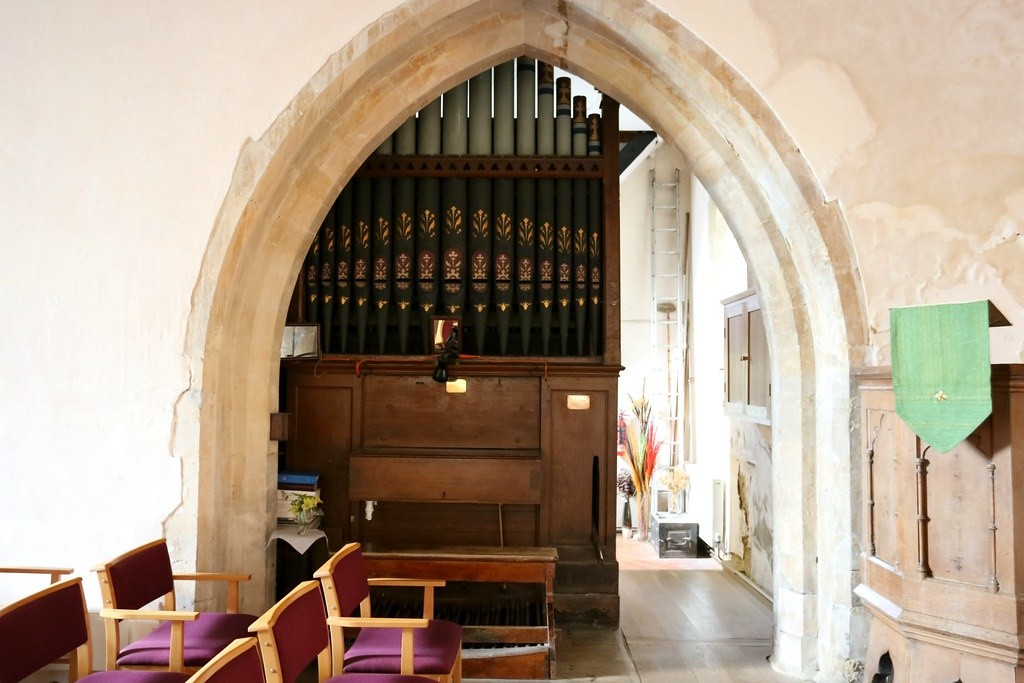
[{"xmin": 276, "ymin": 523, "xmax": 330, "ymax": 605}]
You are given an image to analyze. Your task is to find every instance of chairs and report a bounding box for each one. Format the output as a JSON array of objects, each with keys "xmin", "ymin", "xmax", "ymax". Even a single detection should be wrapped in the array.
[{"xmin": 0, "ymin": 539, "xmax": 464, "ymax": 683}]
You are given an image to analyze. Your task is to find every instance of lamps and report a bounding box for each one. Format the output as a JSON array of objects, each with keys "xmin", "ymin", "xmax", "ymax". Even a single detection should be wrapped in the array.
[{"xmin": 432, "ymin": 325, "xmax": 460, "ymax": 384}]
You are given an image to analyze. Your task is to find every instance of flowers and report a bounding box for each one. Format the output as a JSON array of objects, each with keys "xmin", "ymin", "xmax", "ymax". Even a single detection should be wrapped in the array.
[
  {"xmin": 615, "ymin": 469, "xmax": 638, "ymax": 500},
  {"xmin": 291, "ymin": 493, "xmax": 323, "ymax": 517}
]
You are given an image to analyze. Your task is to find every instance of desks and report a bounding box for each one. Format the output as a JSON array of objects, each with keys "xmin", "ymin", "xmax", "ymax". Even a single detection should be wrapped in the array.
[{"xmin": 329, "ymin": 543, "xmax": 559, "ymax": 680}]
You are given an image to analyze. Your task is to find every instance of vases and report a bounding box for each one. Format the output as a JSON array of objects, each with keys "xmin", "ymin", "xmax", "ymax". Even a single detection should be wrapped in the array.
[
  {"xmin": 298, "ymin": 511, "xmax": 309, "ymax": 536},
  {"xmin": 622, "ymin": 499, "xmax": 632, "ymax": 539},
  {"xmin": 636, "ymin": 491, "xmax": 651, "ymax": 541}
]
